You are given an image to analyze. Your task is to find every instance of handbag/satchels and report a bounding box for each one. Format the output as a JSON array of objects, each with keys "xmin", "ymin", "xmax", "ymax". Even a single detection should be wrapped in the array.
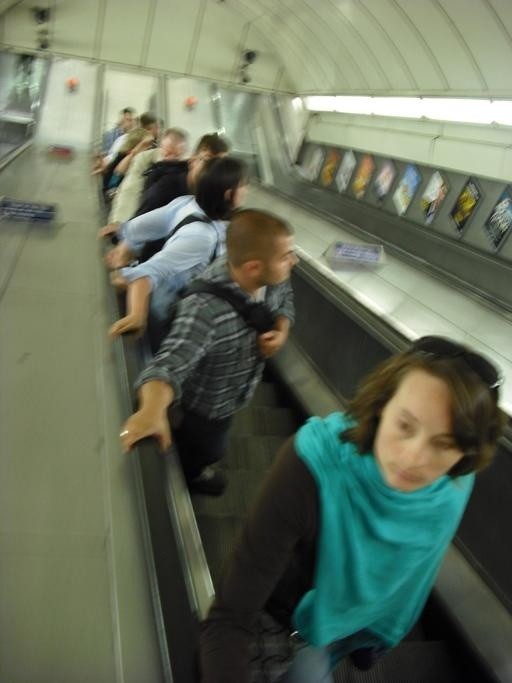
[{"xmin": 249, "ymin": 607, "xmax": 295, "ymax": 683}]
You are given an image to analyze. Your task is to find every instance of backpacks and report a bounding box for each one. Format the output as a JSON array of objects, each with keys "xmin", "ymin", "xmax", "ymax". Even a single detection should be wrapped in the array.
[{"xmin": 144, "ymin": 160, "xmax": 187, "ymax": 201}]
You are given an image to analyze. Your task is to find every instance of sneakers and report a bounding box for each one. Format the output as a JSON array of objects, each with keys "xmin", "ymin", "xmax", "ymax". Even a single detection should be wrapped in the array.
[{"xmin": 199, "ymin": 465, "xmax": 229, "ymax": 497}]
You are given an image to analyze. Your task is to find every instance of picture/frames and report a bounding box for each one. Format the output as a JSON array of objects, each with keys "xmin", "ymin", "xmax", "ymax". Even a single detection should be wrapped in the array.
[{"xmin": 307, "ymin": 144, "xmax": 512, "ymax": 257}]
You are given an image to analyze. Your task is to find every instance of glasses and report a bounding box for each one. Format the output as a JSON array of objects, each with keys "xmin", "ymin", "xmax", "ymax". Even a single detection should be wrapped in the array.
[{"xmin": 414, "ymin": 335, "xmax": 501, "ymax": 403}]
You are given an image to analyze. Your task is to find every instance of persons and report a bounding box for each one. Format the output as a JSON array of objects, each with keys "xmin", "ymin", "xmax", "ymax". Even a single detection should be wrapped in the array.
[
  {"xmin": 117, "ymin": 206, "xmax": 299, "ymax": 501},
  {"xmin": 423, "ymin": 182, "xmax": 448, "ymax": 223},
  {"xmin": 195, "ymin": 333, "xmax": 504, "ymax": 682},
  {"xmin": 90, "ymin": 105, "xmax": 246, "ymax": 355}
]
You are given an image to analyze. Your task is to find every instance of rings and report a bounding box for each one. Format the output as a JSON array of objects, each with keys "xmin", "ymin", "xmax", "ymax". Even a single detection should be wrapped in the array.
[{"xmin": 120, "ymin": 430, "xmax": 129, "ymax": 438}]
